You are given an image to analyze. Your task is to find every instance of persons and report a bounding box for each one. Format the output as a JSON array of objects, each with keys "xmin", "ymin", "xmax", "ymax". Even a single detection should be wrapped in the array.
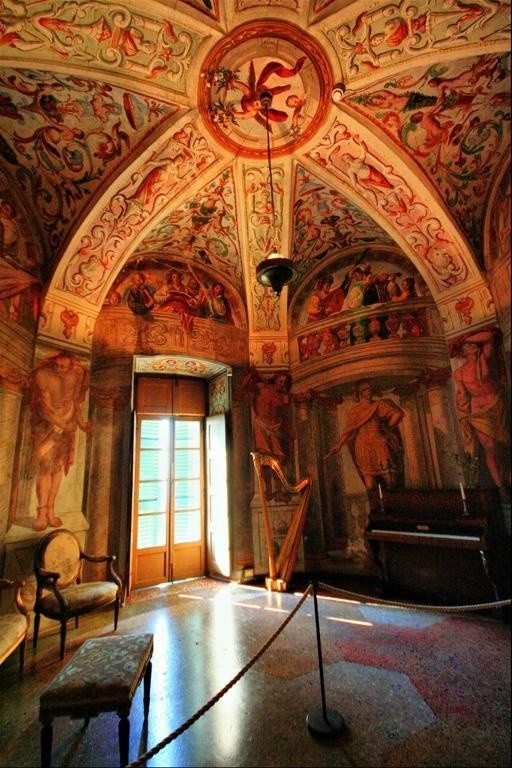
[
  {"xmin": 248, "ymin": 373, "xmax": 290, "ymax": 502},
  {"xmin": 30, "ymin": 351, "xmax": 96, "ymax": 531},
  {"xmin": 454, "ymin": 331, "xmax": 512, "ymax": 497},
  {"xmin": 0, "ymin": 69, "xmax": 512, "ymax": 345},
  {"xmin": 328, "ymin": 387, "xmax": 404, "ymax": 489}
]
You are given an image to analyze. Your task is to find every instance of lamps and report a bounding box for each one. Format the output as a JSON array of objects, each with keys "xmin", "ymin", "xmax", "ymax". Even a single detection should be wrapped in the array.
[{"xmin": 255, "ymin": 90, "xmax": 298, "ymax": 296}]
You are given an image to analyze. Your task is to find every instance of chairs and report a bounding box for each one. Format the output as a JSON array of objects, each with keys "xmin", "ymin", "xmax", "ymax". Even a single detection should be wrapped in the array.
[{"xmin": 0, "ymin": 528, "xmax": 123, "ymax": 670}]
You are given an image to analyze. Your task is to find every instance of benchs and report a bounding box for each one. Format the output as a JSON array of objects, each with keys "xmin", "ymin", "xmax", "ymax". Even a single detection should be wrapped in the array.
[{"xmin": 37, "ymin": 633, "xmax": 153, "ymax": 767}]
